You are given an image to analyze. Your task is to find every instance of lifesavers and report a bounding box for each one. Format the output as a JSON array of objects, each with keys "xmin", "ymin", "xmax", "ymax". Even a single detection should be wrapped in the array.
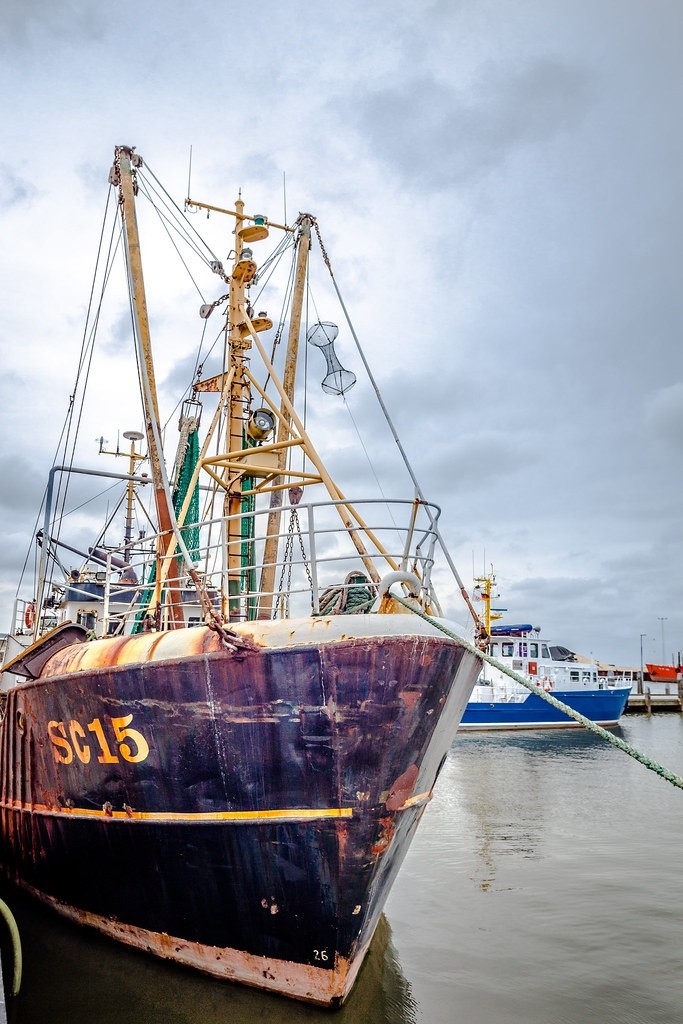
[
  {"xmin": 541, "ymin": 678, "xmax": 552, "ymax": 692},
  {"xmin": 24, "ymin": 605, "xmax": 37, "ymax": 627}
]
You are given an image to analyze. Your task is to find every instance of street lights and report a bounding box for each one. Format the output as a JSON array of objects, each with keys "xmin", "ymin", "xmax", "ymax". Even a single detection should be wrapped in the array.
[
  {"xmin": 656, "ymin": 616, "xmax": 668, "ymax": 667},
  {"xmin": 640, "ymin": 633, "xmax": 647, "ymax": 680}
]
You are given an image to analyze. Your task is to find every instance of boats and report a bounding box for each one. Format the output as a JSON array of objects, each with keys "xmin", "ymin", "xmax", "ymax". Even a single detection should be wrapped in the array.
[
  {"xmin": 453, "ymin": 543, "xmax": 650, "ymax": 737},
  {"xmin": 1, "ymin": 141, "xmax": 491, "ymax": 1005},
  {"xmin": 643, "ymin": 663, "xmax": 683, "ymax": 682}
]
